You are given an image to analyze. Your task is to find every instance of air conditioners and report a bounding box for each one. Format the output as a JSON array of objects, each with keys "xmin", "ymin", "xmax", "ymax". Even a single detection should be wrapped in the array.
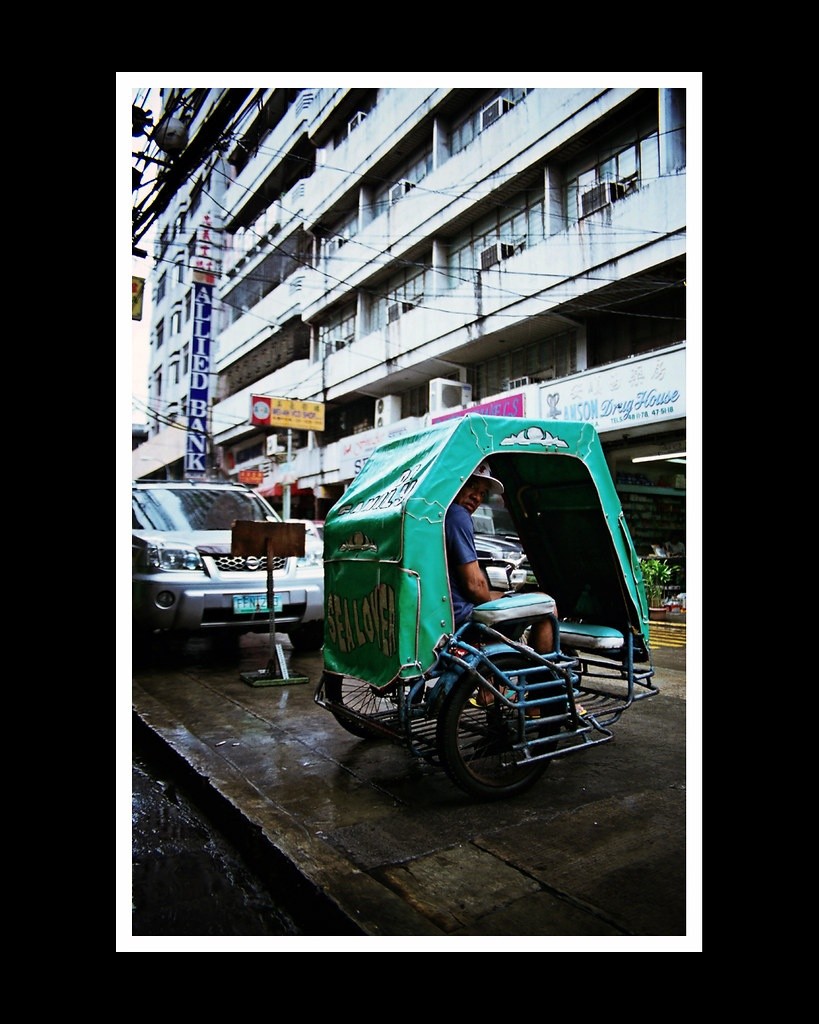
[
  {"xmin": 389, "ymin": 179, "xmax": 417, "ymax": 209},
  {"xmin": 374, "ymin": 395, "xmax": 402, "ymax": 428},
  {"xmin": 480, "ymin": 96, "xmax": 516, "ymax": 135},
  {"xmin": 578, "ymin": 180, "xmax": 625, "ymax": 219},
  {"xmin": 386, "ymin": 300, "xmax": 417, "ymax": 327},
  {"xmin": 348, "ymin": 111, "xmax": 368, "ymax": 135},
  {"xmin": 508, "ymin": 376, "xmax": 540, "ymax": 390},
  {"xmin": 324, "ymin": 339, "xmax": 349, "ymax": 358},
  {"xmin": 429, "ymin": 377, "xmax": 472, "ymax": 414},
  {"xmin": 325, "ymin": 235, "xmax": 347, "ymax": 258},
  {"xmin": 477, "ymin": 241, "xmax": 514, "ymax": 270}
]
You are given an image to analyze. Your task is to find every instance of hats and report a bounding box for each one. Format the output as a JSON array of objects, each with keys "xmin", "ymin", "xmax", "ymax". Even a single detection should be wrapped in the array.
[{"xmin": 472, "ymin": 460, "xmax": 505, "ymax": 494}]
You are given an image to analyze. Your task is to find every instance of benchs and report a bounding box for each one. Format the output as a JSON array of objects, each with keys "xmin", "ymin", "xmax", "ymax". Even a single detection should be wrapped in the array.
[{"xmin": 556, "ymin": 620, "xmax": 625, "ymax": 650}]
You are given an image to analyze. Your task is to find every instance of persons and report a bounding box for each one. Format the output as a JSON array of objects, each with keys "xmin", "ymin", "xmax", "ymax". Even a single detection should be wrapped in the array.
[
  {"xmin": 665, "ymin": 533, "xmax": 685, "ymax": 583},
  {"xmin": 446, "ymin": 462, "xmax": 586, "ymax": 717}
]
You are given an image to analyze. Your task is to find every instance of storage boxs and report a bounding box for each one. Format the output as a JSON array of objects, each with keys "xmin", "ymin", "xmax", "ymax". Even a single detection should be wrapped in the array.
[{"xmin": 668, "ymin": 473, "xmax": 686, "ymax": 489}]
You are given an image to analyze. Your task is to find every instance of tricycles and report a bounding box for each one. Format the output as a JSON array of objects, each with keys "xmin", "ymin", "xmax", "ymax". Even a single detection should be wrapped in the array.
[{"xmin": 313, "ymin": 415, "xmax": 662, "ymax": 794}]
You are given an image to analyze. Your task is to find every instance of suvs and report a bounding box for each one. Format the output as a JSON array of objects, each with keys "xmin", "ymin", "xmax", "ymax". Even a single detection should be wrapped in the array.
[{"xmin": 133, "ymin": 480, "xmax": 324, "ymax": 666}]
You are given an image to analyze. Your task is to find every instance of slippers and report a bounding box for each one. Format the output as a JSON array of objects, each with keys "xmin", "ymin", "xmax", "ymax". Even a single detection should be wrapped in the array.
[
  {"xmin": 524, "ymin": 704, "xmax": 587, "ymax": 719},
  {"xmin": 469, "ymin": 690, "xmax": 516, "ymax": 708}
]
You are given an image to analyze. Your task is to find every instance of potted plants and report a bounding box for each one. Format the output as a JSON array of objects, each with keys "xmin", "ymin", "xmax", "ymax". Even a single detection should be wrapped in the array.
[{"xmin": 639, "ymin": 559, "xmax": 682, "ymax": 621}]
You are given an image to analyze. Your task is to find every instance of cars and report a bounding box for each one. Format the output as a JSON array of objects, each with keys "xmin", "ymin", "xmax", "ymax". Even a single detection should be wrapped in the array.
[{"xmin": 473, "ymin": 534, "xmax": 527, "ymax": 591}]
